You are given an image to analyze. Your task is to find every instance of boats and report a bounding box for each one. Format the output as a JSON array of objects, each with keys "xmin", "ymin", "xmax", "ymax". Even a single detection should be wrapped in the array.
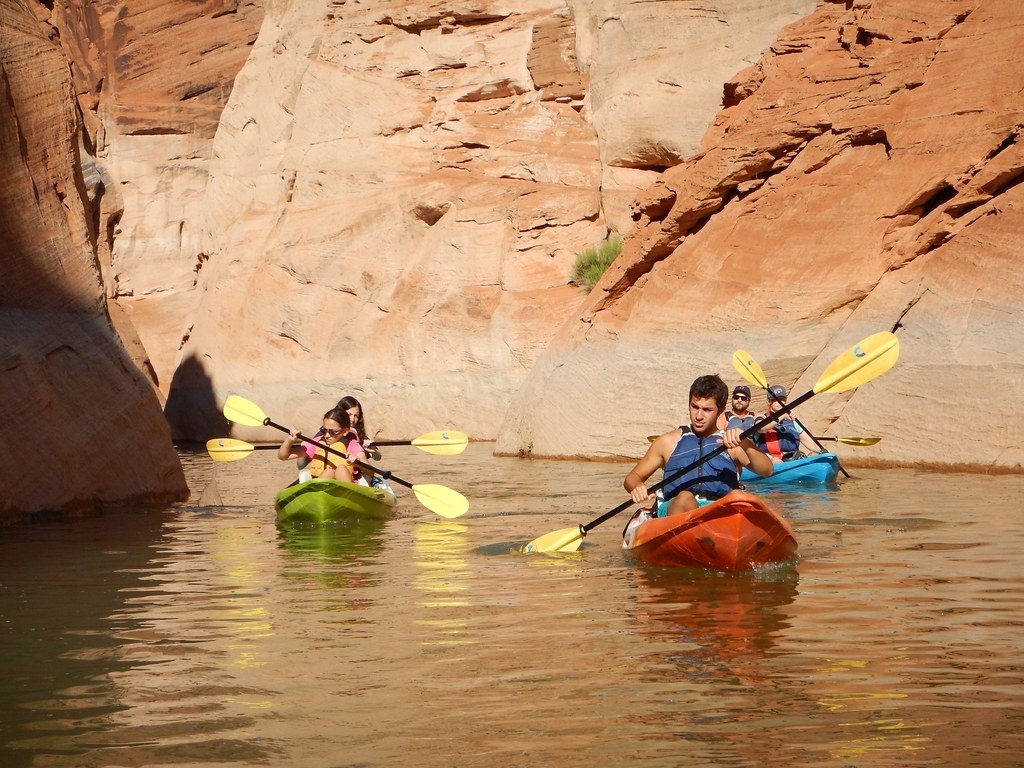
[
  {"xmin": 274, "ymin": 475, "xmax": 397, "ymax": 525},
  {"xmin": 622, "ymin": 488, "xmax": 799, "ymax": 575},
  {"xmin": 738, "ymin": 453, "xmax": 841, "ymax": 485}
]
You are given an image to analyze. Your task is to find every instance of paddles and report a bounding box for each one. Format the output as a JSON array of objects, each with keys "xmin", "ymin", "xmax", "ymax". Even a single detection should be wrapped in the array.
[
  {"xmin": 811, "ymin": 433, "xmax": 883, "ymax": 450},
  {"xmin": 729, "ymin": 346, "xmax": 858, "ymax": 483},
  {"xmin": 521, "ymin": 333, "xmax": 902, "ymax": 556},
  {"xmin": 215, "ymin": 394, "xmax": 474, "ymax": 522},
  {"xmin": 202, "ymin": 427, "xmax": 471, "ymax": 464}
]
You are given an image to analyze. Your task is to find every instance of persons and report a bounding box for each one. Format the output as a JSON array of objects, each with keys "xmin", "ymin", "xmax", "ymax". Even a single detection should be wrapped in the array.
[
  {"xmin": 312, "ymin": 396, "xmax": 382, "ymax": 461},
  {"xmin": 278, "ymin": 409, "xmax": 374, "ymax": 482},
  {"xmin": 753, "ymin": 385, "xmax": 806, "ymax": 464},
  {"xmin": 717, "ymin": 386, "xmax": 759, "ymax": 430},
  {"xmin": 624, "ymin": 372, "xmax": 773, "ymax": 518}
]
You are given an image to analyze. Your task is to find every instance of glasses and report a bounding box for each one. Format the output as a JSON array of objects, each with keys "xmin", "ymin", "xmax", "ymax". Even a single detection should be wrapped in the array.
[
  {"xmin": 320, "ymin": 426, "xmax": 346, "ymax": 437},
  {"xmin": 733, "ymin": 395, "xmax": 747, "ymax": 401}
]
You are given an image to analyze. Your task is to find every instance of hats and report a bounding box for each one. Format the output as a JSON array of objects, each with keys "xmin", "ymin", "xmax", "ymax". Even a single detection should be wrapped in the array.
[
  {"xmin": 767, "ymin": 385, "xmax": 787, "ymax": 400},
  {"xmin": 733, "ymin": 386, "xmax": 751, "ymax": 400}
]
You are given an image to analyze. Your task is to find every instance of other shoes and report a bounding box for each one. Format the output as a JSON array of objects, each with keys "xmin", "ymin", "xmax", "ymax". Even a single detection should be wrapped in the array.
[
  {"xmin": 792, "ymin": 450, "xmax": 807, "ymax": 460},
  {"xmin": 819, "ymin": 449, "xmax": 828, "ymax": 454}
]
